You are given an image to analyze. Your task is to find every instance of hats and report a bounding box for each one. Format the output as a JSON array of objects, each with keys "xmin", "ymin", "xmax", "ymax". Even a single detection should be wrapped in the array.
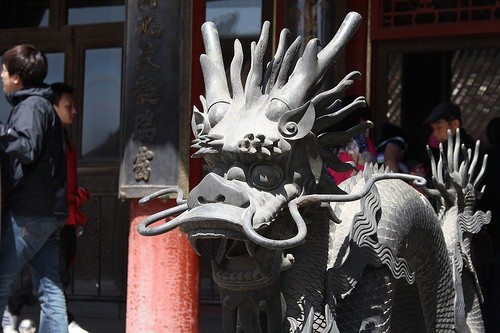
[
  {"xmin": 388, "ymin": 136, "xmax": 406, "ymax": 151},
  {"xmin": 423, "ymin": 101, "xmax": 460, "ymax": 124}
]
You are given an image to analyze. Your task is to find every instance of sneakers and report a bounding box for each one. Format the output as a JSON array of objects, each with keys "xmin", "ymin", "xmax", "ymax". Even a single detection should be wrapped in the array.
[
  {"xmin": 66, "ymin": 321, "xmax": 89, "ymax": 333},
  {"xmin": 2, "ymin": 305, "xmax": 17, "ymax": 332}
]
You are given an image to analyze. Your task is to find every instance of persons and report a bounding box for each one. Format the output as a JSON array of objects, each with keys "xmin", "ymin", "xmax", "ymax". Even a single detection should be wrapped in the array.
[
  {"xmin": 329, "ymin": 98, "xmax": 499, "ymax": 332},
  {"xmin": 0, "ymin": 43, "xmax": 70, "ymax": 332},
  {"xmin": 1, "ymin": 81, "xmax": 92, "ymax": 331}
]
